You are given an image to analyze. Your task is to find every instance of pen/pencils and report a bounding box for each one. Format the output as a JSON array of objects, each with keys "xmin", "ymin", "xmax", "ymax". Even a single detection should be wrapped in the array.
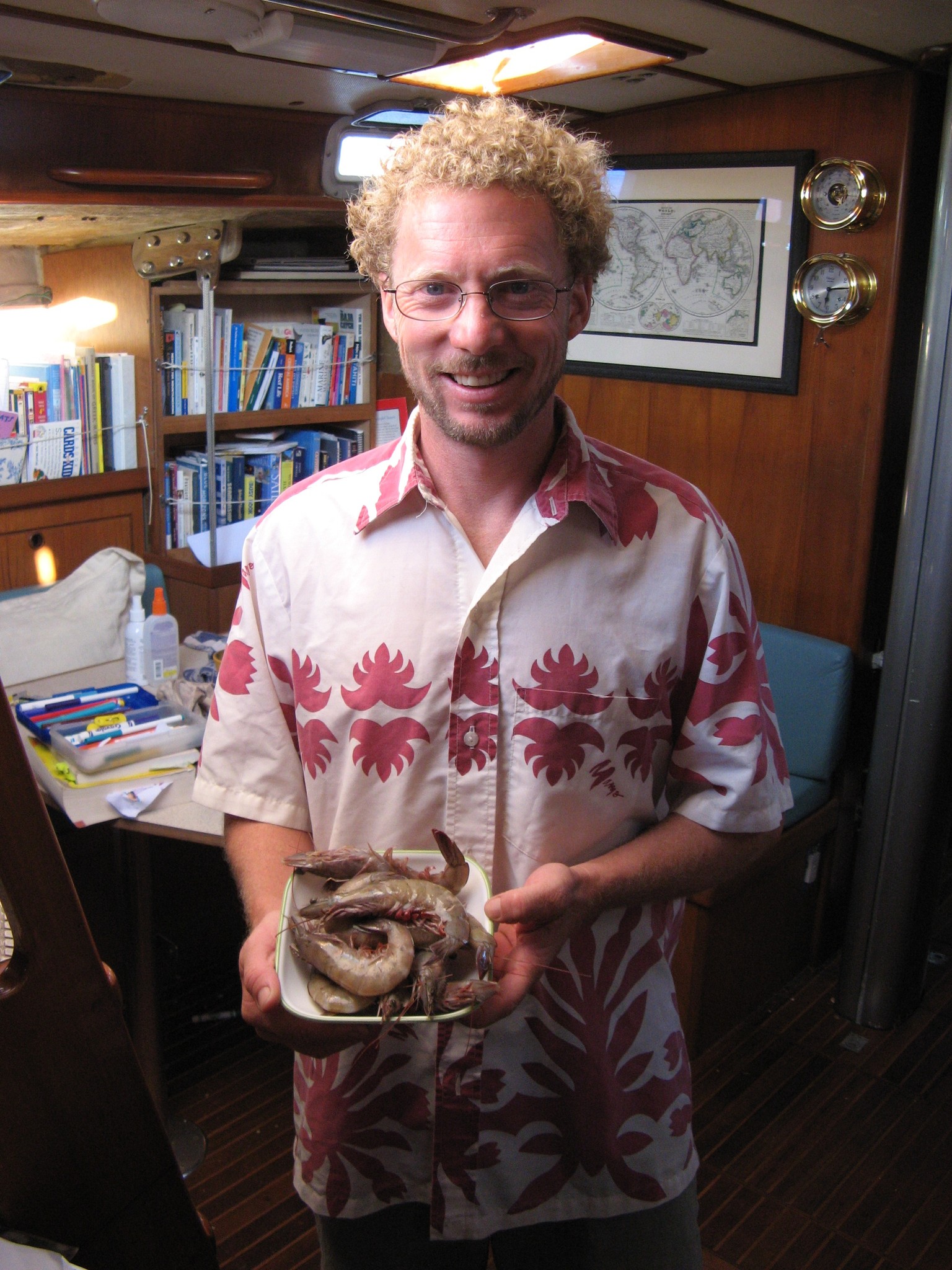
[
  {"xmin": 85, "ymin": 713, "xmax": 184, "ymax": 744},
  {"xmin": 27, "ymin": 698, "xmax": 122, "ymax": 723},
  {"xmin": 44, "ymin": 685, "xmax": 141, "ymax": 711},
  {"xmin": 34, "ymin": 702, "xmax": 116, "ymax": 727}
]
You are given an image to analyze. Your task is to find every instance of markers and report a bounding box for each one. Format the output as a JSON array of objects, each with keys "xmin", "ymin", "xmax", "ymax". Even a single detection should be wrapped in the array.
[
  {"xmin": 17, "ymin": 689, "xmax": 98, "ymax": 713},
  {"xmin": 62, "ymin": 712, "xmax": 160, "ymax": 746},
  {"xmin": 81, "ymin": 726, "xmax": 173, "ymax": 751}
]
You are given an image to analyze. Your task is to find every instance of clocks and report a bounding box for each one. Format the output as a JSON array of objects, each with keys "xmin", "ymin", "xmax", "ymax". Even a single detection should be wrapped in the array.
[{"xmin": 793, "ymin": 257, "xmax": 882, "ymax": 344}]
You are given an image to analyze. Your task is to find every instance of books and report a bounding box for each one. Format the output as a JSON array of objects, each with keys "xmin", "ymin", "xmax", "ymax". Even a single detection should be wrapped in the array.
[{"xmin": 0, "ymin": 302, "xmax": 364, "ymax": 551}]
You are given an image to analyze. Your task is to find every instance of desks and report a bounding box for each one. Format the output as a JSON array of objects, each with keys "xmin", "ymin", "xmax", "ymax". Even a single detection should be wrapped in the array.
[{"xmin": 3, "ymin": 635, "xmax": 397, "ymax": 1132}]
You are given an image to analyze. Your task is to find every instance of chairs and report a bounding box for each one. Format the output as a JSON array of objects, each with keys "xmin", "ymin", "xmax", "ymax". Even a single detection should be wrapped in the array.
[{"xmin": 674, "ymin": 622, "xmax": 857, "ymax": 1062}]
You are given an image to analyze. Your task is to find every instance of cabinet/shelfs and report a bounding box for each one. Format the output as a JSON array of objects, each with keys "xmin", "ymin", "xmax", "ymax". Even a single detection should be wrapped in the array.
[
  {"xmin": 153, "ymin": 278, "xmax": 377, "ymax": 644},
  {"xmin": 0, "ymin": 464, "xmax": 156, "ymax": 595}
]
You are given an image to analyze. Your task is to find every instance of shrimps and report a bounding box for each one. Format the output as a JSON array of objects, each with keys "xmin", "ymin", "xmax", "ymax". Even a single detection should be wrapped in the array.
[{"xmin": 282, "ymin": 827, "xmax": 502, "ymax": 1022}]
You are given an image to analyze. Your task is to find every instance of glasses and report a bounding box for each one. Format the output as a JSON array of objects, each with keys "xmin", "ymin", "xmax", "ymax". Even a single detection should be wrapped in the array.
[{"xmin": 382, "ymin": 270, "xmax": 582, "ymax": 322}]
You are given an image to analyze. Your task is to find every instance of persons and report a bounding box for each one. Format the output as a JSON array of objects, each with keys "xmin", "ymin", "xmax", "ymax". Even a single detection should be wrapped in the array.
[{"xmin": 191, "ymin": 98, "xmax": 795, "ymax": 1270}]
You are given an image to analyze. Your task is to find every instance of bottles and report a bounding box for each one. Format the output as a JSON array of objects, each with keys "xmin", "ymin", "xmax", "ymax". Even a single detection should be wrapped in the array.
[
  {"xmin": 123, "ymin": 595, "xmax": 148, "ymax": 687},
  {"xmin": 143, "ymin": 587, "xmax": 181, "ymax": 688}
]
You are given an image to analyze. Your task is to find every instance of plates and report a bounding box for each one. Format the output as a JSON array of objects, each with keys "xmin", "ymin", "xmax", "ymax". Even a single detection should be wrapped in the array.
[{"xmin": 274, "ymin": 849, "xmax": 494, "ymax": 1026}]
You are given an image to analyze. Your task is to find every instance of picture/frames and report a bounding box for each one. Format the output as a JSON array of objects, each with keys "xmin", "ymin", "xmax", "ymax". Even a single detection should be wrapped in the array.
[{"xmin": 562, "ymin": 148, "xmax": 817, "ymax": 396}]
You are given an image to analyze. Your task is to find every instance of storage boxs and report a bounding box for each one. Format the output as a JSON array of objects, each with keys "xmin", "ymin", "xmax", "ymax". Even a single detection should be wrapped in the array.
[{"xmin": 16, "ymin": 682, "xmax": 208, "ymax": 773}]
